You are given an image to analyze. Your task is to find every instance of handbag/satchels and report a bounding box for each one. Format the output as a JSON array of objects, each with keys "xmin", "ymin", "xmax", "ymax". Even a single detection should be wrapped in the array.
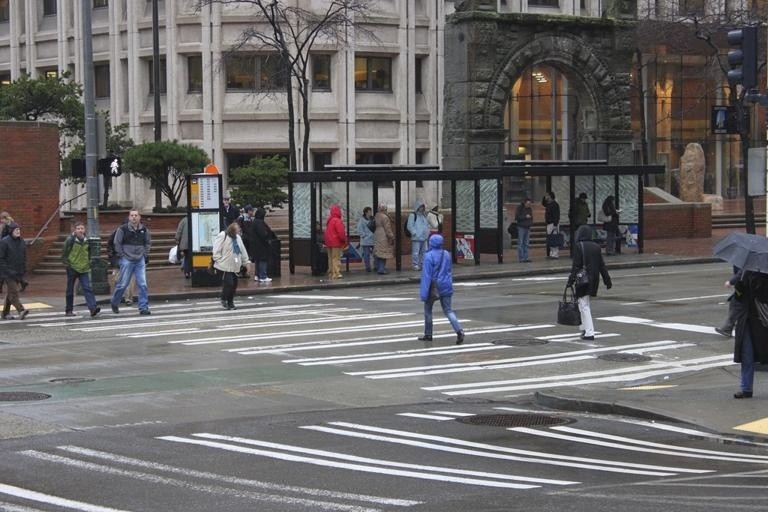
[
  {"xmin": 206, "ymin": 257, "xmax": 224, "ymax": 282},
  {"xmin": 366, "ymin": 216, "xmax": 376, "ymax": 234},
  {"xmin": 557, "ymin": 284, "xmax": 581, "ymax": 327},
  {"xmin": 597, "ymin": 209, "xmax": 612, "ymax": 223},
  {"xmin": 754, "ymin": 296, "xmax": 768, "ymax": 328},
  {"xmin": 573, "ymin": 269, "xmax": 591, "ymax": 290},
  {"xmin": 424, "ymin": 281, "xmax": 440, "ymax": 305},
  {"xmin": 168, "ymin": 243, "xmax": 183, "ymax": 264},
  {"xmin": 546, "ymin": 228, "xmax": 565, "ymax": 247},
  {"xmin": 507, "ymin": 222, "xmax": 520, "ymax": 239}
]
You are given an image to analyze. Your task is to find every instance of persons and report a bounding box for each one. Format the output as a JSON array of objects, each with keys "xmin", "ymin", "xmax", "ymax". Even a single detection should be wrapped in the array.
[
  {"xmin": 732, "ymin": 262, "xmax": 768, "ymax": 399},
  {"xmin": 60, "ymin": 220, "xmax": 102, "ymax": 317},
  {"xmin": 713, "ymin": 268, "xmax": 767, "ymax": 337},
  {"xmin": 601, "ymin": 194, "xmax": 620, "ymax": 256},
  {"xmin": 566, "ymin": 223, "xmax": 612, "ymax": 341},
  {"xmin": 110, "ymin": 208, "xmax": 152, "ymax": 317},
  {"xmin": 417, "ymin": 234, "xmax": 465, "ymax": 345},
  {"xmin": 314, "ymin": 200, "xmax": 444, "ymax": 280},
  {"xmin": 567, "ymin": 191, "xmax": 591, "ymax": 255},
  {"xmin": 105, "ymin": 218, "xmax": 134, "ymax": 306},
  {"xmin": 513, "ymin": 197, "xmax": 535, "ymax": 262},
  {"xmin": 0, "ymin": 220, "xmax": 30, "ymax": 320},
  {"xmin": 211, "ymin": 222, "xmax": 252, "ymax": 310},
  {"xmin": 222, "ymin": 193, "xmax": 276, "ymax": 281},
  {"xmin": 0, "ymin": 210, "xmax": 28, "ymax": 294},
  {"xmin": 541, "ymin": 191, "xmax": 562, "ymax": 260},
  {"xmin": 175, "ymin": 214, "xmax": 189, "ymax": 280}
]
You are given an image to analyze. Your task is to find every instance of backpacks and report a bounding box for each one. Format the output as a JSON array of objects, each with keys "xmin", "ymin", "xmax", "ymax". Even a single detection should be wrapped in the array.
[{"xmin": 404, "ymin": 212, "xmax": 417, "ymax": 238}]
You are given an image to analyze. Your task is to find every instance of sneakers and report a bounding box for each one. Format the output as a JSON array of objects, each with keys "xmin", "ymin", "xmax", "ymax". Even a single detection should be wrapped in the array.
[
  {"xmin": 328, "ymin": 272, "xmax": 344, "ymax": 280},
  {"xmin": 519, "ymin": 259, "xmax": 533, "ymax": 263},
  {"xmin": 412, "ymin": 264, "xmax": 422, "ymax": 271},
  {"xmin": 66, "ymin": 311, "xmax": 76, "ymax": 316},
  {"xmin": 259, "ymin": 277, "xmax": 273, "ymax": 282},
  {"xmin": 254, "ymin": 275, "xmax": 260, "ymax": 281},
  {"xmin": 365, "ymin": 266, "xmax": 391, "ymax": 276},
  {"xmin": 579, "ymin": 330, "xmax": 595, "ymax": 341},
  {"xmin": 220, "ymin": 300, "xmax": 239, "ymax": 310},
  {"xmin": 125, "ymin": 301, "xmax": 134, "ymax": 307},
  {"xmin": 715, "ymin": 327, "xmax": 732, "ymax": 338},
  {"xmin": 91, "ymin": 307, "xmax": 100, "ymax": 317},
  {"xmin": 19, "ymin": 309, "xmax": 29, "ymax": 320},
  {"xmin": 1, "ymin": 313, "xmax": 15, "ymax": 320},
  {"xmin": 456, "ymin": 329, "xmax": 464, "ymax": 345},
  {"xmin": 18, "ymin": 280, "xmax": 29, "ymax": 292},
  {"xmin": 544, "ymin": 256, "xmax": 560, "ymax": 261},
  {"xmin": 733, "ymin": 390, "xmax": 753, "ymax": 399},
  {"xmin": 418, "ymin": 335, "xmax": 432, "ymax": 341},
  {"xmin": 111, "ymin": 303, "xmax": 119, "ymax": 313},
  {"xmin": 140, "ymin": 309, "xmax": 151, "ymax": 315}
]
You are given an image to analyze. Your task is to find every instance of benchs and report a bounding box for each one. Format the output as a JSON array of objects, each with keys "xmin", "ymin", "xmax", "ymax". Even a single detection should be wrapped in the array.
[
  {"xmin": 558, "ymin": 223, "xmax": 637, "ymax": 251},
  {"xmin": 430, "ymin": 230, "xmax": 474, "ymax": 259},
  {"xmin": 341, "ymin": 242, "xmax": 362, "ymax": 263}
]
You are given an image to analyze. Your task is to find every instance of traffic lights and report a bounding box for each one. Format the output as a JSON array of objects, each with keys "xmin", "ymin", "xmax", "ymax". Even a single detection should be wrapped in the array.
[
  {"xmin": 73, "ymin": 159, "xmax": 83, "ymax": 176},
  {"xmin": 726, "ymin": 26, "xmax": 758, "ymax": 89},
  {"xmin": 101, "ymin": 156, "xmax": 122, "ymax": 178}
]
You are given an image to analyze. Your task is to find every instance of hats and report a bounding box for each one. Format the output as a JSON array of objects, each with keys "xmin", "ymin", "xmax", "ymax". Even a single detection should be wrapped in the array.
[
  {"xmin": 7, "ymin": 221, "xmax": 22, "ymax": 236},
  {"xmin": 579, "ymin": 192, "xmax": 589, "ymax": 199},
  {"xmin": 244, "ymin": 204, "xmax": 257, "ymax": 211}
]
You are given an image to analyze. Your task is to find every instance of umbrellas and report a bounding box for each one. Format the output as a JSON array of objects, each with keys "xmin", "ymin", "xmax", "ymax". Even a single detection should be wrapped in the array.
[{"xmin": 709, "ymin": 231, "xmax": 768, "ymax": 275}]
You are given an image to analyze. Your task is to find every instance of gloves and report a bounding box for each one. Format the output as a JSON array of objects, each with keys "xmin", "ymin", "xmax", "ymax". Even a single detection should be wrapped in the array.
[{"xmin": 604, "ymin": 278, "xmax": 612, "ymax": 290}]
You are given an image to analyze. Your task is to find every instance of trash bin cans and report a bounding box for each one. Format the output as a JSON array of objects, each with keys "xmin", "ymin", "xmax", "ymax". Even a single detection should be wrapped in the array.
[
  {"xmin": 268, "ymin": 239, "xmax": 280, "ymax": 276},
  {"xmin": 92, "ymin": 256, "xmax": 110, "ymax": 295}
]
